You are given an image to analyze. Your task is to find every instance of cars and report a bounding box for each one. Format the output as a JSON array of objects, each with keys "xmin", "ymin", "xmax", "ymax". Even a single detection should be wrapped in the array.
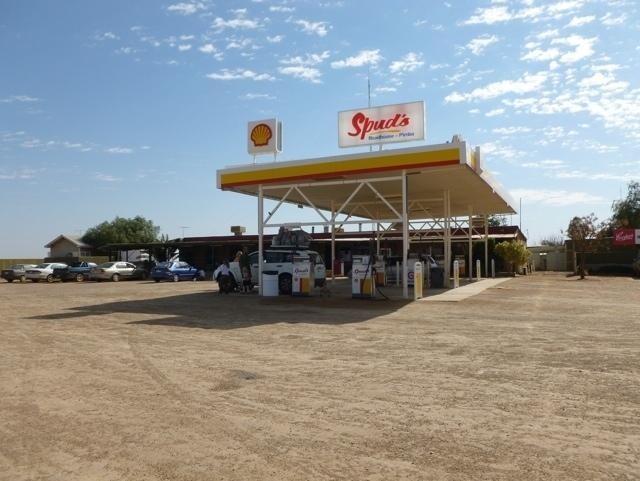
[
  {"xmin": 0, "ymin": 263, "xmax": 39, "ymax": 283},
  {"xmin": 24, "ymin": 262, "xmax": 68, "ymax": 283},
  {"xmin": 90, "ymin": 260, "xmax": 149, "ymax": 282},
  {"xmin": 149, "ymin": 260, "xmax": 208, "ymax": 282}
]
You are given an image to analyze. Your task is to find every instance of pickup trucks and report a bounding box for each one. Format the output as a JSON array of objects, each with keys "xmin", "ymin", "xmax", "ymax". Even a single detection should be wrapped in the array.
[{"xmin": 53, "ymin": 260, "xmax": 98, "ymax": 282}]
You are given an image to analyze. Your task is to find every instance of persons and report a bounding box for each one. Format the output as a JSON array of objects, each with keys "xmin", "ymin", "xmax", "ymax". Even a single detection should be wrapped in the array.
[
  {"xmin": 239, "ymin": 246, "xmax": 252, "ymax": 281},
  {"xmin": 216, "ymin": 258, "xmax": 230, "ymax": 295},
  {"xmin": 234, "ymin": 250, "xmax": 244, "ymax": 262}
]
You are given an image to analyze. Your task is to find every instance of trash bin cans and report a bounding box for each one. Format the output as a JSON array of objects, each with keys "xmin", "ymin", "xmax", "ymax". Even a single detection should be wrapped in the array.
[{"xmin": 262, "ymin": 271, "xmax": 279, "ymax": 296}]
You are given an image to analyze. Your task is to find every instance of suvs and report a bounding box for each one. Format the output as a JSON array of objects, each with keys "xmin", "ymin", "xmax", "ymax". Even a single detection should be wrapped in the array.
[
  {"xmin": 211, "ymin": 244, "xmax": 327, "ymax": 294},
  {"xmin": 384, "ymin": 254, "xmax": 439, "ymax": 281}
]
